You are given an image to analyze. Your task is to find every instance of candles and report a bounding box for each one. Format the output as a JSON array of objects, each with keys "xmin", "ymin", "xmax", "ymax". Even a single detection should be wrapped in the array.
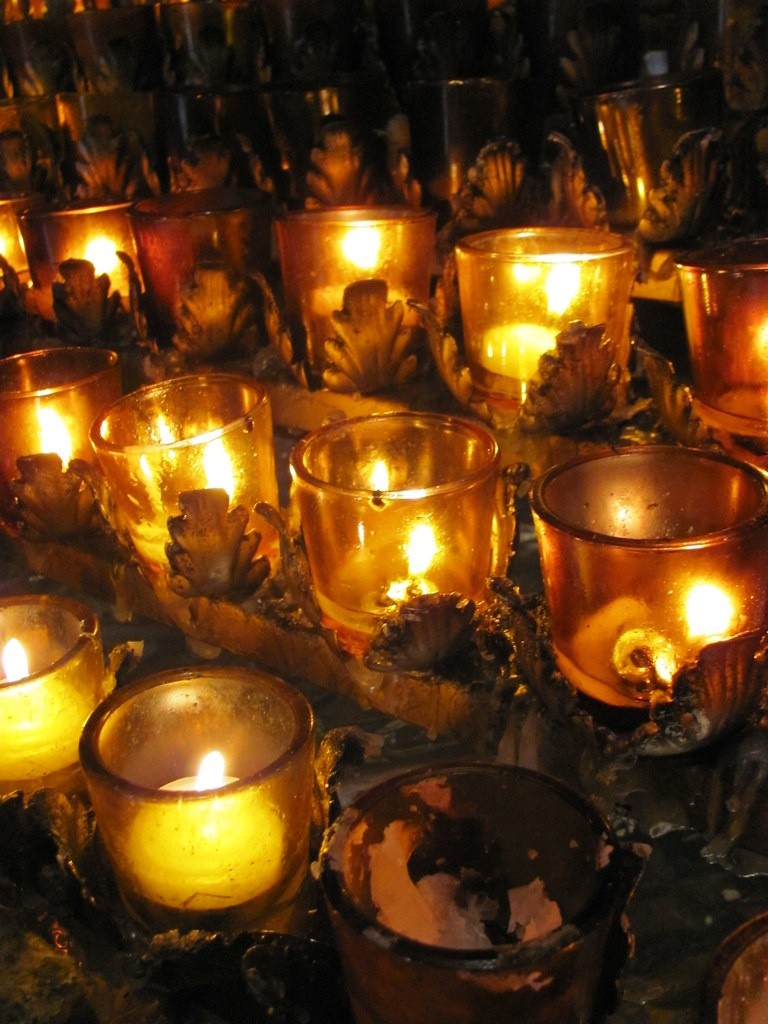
[
  {"xmin": 80, "ymin": 671, "xmax": 312, "ymax": 929},
  {"xmin": 529, "ymin": 452, "xmax": 767, "ymax": 703},
  {"xmin": 0, "ymin": 195, "xmax": 34, "ymax": 290},
  {"xmin": 285, "ymin": 417, "xmax": 496, "ymax": 634},
  {"xmin": 0, "ymin": 350, "xmax": 119, "ymax": 469},
  {"xmin": 277, "ymin": 209, "xmax": 437, "ymax": 373},
  {"xmin": 0, "ymin": 594, "xmax": 121, "ymax": 788},
  {"xmin": 88, "ymin": 373, "xmax": 277, "ymax": 570},
  {"xmin": 457, "ymin": 230, "xmax": 632, "ymax": 394},
  {"xmin": 46, "ymin": 200, "xmax": 140, "ymax": 303},
  {"xmin": 674, "ymin": 241, "xmax": 768, "ymax": 439}
]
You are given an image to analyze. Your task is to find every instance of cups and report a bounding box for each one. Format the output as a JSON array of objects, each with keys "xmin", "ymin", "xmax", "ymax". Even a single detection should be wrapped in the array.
[{"xmin": 0, "ymin": 191, "xmax": 768, "ymax": 1024}]
